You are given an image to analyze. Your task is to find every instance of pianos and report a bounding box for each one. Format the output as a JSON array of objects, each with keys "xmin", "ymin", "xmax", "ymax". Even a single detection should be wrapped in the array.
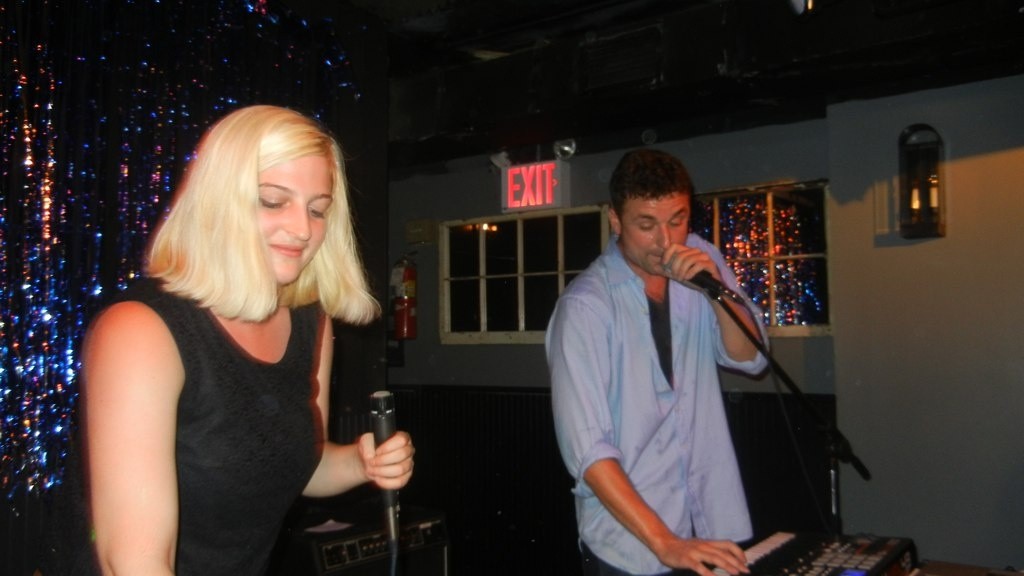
[{"xmin": 654, "ymin": 527, "xmax": 923, "ymax": 576}]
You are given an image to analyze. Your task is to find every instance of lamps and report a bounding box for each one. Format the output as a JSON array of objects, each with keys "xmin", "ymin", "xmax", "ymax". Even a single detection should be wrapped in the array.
[{"xmin": 488, "ymin": 139, "xmax": 577, "ymax": 213}]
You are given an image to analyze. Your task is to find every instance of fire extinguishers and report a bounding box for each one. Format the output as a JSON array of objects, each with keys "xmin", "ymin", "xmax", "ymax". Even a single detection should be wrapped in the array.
[{"xmin": 390, "ymin": 250, "xmax": 418, "ymax": 339}]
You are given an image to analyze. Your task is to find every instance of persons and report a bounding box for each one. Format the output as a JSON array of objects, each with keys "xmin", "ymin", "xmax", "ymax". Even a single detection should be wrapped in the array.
[
  {"xmin": 54, "ymin": 105, "xmax": 416, "ymax": 576},
  {"xmin": 542, "ymin": 149, "xmax": 771, "ymax": 576}
]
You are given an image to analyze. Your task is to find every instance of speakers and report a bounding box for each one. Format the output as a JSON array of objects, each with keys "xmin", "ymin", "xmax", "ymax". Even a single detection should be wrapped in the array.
[{"xmin": 297, "ymin": 514, "xmax": 449, "ymax": 576}]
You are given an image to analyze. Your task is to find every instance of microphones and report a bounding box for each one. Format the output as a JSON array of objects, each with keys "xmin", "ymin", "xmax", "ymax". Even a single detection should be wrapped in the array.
[
  {"xmin": 370, "ymin": 389, "xmax": 401, "ymax": 542},
  {"xmin": 661, "ymin": 254, "xmax": 745, "ymax": 305}
]
